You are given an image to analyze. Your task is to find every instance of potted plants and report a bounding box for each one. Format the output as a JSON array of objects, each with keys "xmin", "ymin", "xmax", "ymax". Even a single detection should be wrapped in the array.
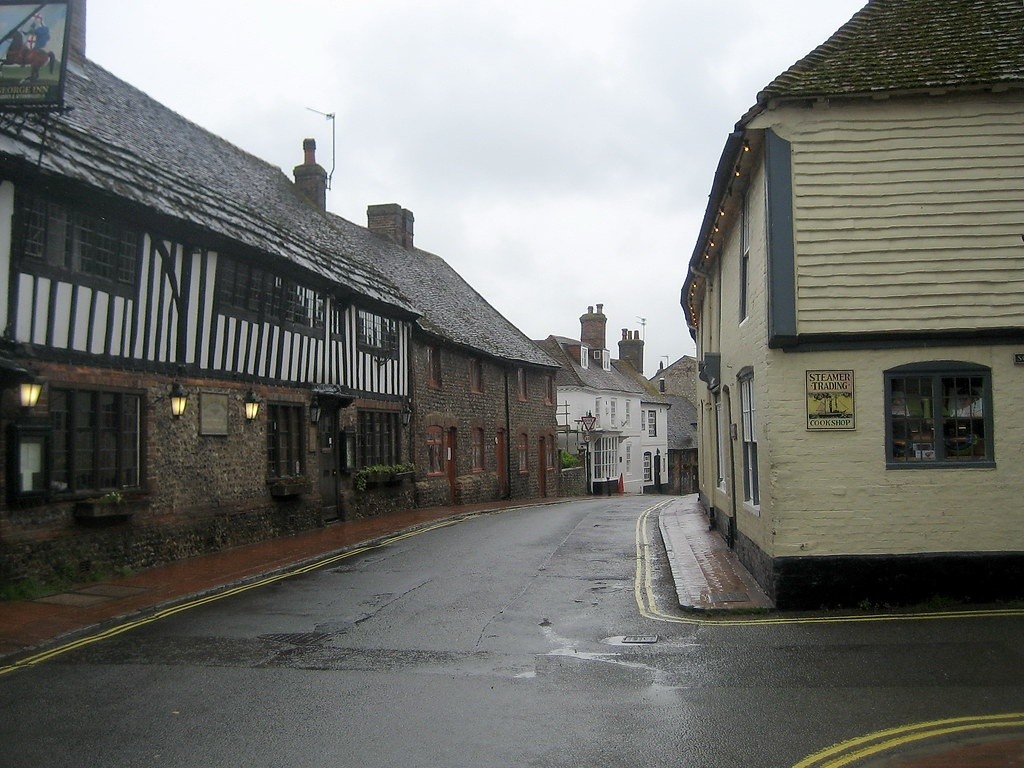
[
  {"xmin": 356, "ymin": 463, "xmax": 417, "ymax": 492},
  {"xmin": 271, "ymin": 475, "xmax": 314, "ymax": 496},
  {"xmin": 74, "ymin": 491, "xmax": 146, "ymax": 518}
]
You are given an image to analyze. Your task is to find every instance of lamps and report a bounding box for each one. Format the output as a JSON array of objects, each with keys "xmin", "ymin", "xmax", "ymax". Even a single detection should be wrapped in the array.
[
  {"xmin": 308, "ymin": 391, "xmax": 322, "ymax": 424},
  {"xmin": 168, "ymin": 364, "xmax": 189, "ymax": 417},
  {"xmin": 18, "ymin": 359, "xmax": 46, "ymax": 409},
  {"xmin": 243, "ymin": 387, "xmax": 262, "ymax": 421},
  {"xmin": 402, "ymin": 399, "xmax": 412, "ymax": 426}
]
